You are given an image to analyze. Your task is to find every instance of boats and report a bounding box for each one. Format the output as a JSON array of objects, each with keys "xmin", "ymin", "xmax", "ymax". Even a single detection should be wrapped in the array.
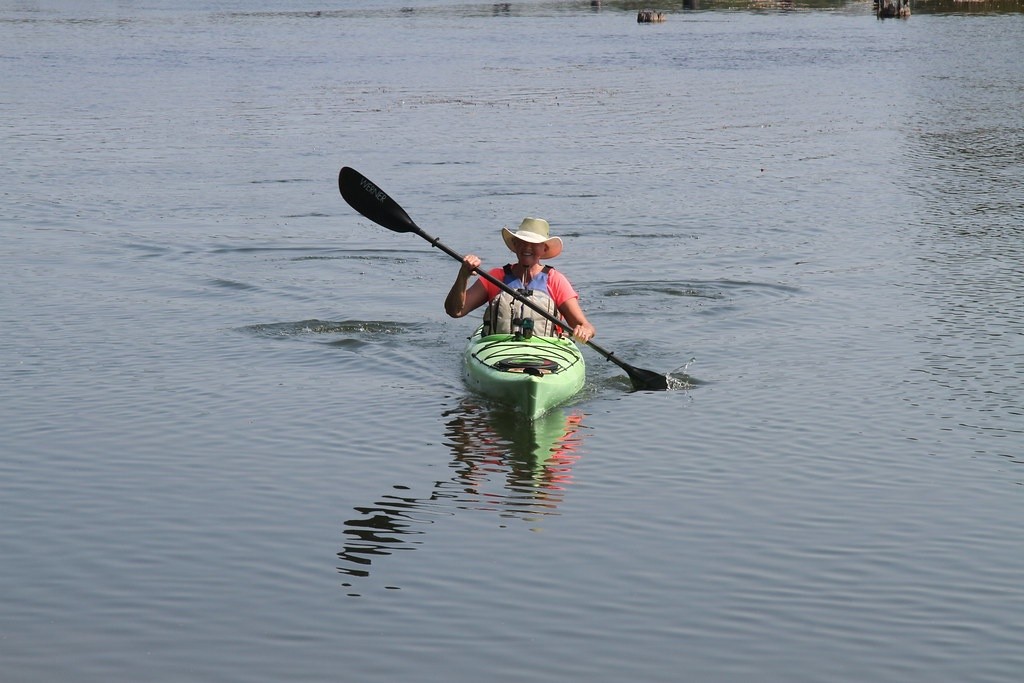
[{"xmin": 462, "ymin": 324, "xmax": 586, "ymax": 419}]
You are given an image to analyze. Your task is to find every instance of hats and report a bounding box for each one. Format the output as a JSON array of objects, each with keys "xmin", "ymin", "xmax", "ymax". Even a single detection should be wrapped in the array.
[{"xmin": 502, "ymin": 217, "xmax": 563, "ymax": 259}]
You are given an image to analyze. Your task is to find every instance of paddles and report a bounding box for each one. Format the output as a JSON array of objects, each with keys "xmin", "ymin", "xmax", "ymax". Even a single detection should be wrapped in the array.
[{"xmin": 335, "ymin": 163, "xmax": 669, "ymax": 394}]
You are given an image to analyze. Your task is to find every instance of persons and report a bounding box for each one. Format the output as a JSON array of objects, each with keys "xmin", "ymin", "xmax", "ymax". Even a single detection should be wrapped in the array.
[{"xmin": 444, "ymin": 218, "xmax": 595, "ymax": 345}]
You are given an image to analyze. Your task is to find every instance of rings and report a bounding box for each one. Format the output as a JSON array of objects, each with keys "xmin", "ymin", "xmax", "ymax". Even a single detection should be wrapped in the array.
[{"xmin": 583, "ymin": 333, "xmax": 586, "ymax": 336}]
